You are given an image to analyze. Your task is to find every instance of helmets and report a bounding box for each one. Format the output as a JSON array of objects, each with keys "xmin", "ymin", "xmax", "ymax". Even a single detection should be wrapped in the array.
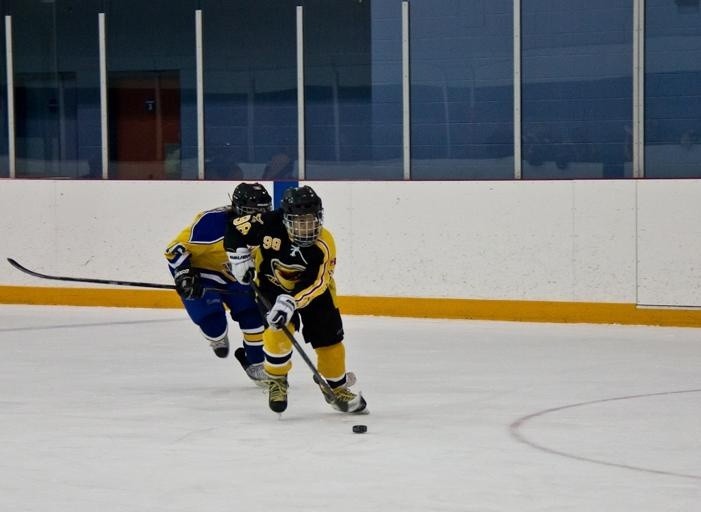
[
  {"xmin": 281, "ymin": 185, "xmax": 324, "ymax": 248},
  {"xmin": 231, "ymin": 182, "xmax": 273, "ymax": 217}
]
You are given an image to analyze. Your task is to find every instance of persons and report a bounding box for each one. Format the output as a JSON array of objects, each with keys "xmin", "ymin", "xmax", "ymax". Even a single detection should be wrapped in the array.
[
  {"xmin": 223, "ymin": 184, "xmax": 368, "ymax": 413},
  {"xmin": 163, "ymin": 182, "xmax": 273, "ymax": 386}
]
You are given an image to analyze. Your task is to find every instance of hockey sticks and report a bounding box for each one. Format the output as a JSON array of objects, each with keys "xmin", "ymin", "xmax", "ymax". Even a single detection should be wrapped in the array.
[
  {"xmin": 246, "ymin": 272, "xmax": 363, "ymax": 411},
  {"xmin": 7, "ymin": 258, "xmax": 178, "ymax": 289}
]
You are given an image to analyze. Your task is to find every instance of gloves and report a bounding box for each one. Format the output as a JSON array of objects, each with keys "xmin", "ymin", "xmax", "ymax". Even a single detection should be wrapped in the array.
[
  {"xmin": 226, "ymin": 246, "xmax": 257, "ymax": 285},
  {"xmin": 174, "ymin": 268, "xmax": 207, "ymax": 301}
]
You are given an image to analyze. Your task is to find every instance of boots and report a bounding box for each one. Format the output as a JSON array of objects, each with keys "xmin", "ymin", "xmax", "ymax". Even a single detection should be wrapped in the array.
[
  {"xmin": 268, "ymin": 377, "xmax": 287, "ymax": 413},
  {"xmin": 265, "ymin": 294, "xmax": 297, "ymax": 333}
]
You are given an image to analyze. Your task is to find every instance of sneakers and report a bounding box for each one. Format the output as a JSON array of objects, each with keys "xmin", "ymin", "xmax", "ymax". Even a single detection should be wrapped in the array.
[
  {"xmin": 208, "ymin": 335, "xmax": 229, "ymax": 358},
  {"xmin": 320, "ymin": 387, "xmax": 367, "ymax": 411},
  {"xmin": 235, "ymin": 348, "xmax": 269, "ymax": 380}
]
